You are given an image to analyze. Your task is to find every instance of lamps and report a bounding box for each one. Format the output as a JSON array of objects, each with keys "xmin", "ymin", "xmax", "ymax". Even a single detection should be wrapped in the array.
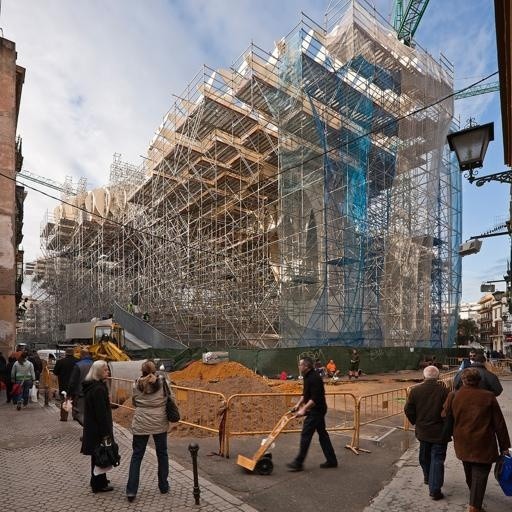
[
  {"xmin": 492, "ymin": 290, "xmax": 512, "ymax": 306},
  {"xmin": 447, "ymin": 117, "xmax": 512, "ymax": 188}
]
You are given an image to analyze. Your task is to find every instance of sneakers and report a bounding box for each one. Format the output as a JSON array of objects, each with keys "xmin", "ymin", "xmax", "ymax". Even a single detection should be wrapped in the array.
[
  {"xmin": 466, "ymin": 504, "xmax": 486, "ymax": 511},
  {"xmin": 287, "ymin": 462, "xmax": 303, "ymax": 469},
  {"xmin": 320, "ymin": 461, "xmax": 338, "ymax": 468},
  {"xmin": 429, "ymin": 489, "xmax": 443, "ymax": 498},
  {"xmin": 6, "ymin": 398, "xmax": 28, "ymax": 410}
]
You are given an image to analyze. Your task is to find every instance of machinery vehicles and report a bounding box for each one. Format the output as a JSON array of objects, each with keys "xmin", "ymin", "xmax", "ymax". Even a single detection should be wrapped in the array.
[{"xmin": 55, "ymin": 314, "xmax": 132, "ymax": 364}]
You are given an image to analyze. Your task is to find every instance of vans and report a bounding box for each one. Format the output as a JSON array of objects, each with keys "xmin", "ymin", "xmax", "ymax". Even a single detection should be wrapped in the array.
[{"xmin": 36, "ymin": 348, "xmax": 64, "ymax": 367}]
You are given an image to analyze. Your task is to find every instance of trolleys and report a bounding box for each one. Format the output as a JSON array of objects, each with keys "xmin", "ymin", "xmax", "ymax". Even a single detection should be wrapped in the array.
[{"xmin": 236, "ymin": 408, "xmax": 304, "ymax": 477}]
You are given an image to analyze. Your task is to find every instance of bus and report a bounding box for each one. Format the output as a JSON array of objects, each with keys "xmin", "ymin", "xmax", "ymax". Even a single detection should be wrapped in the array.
[{"xmin": 16, "ymin": 342, "xmax": 30, "ymax": 358}]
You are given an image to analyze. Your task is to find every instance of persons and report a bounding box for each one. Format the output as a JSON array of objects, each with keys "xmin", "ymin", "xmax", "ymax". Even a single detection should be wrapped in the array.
[
  {"xmin": 404, "ymin": 348, "xmax": 512, "ymax": 512},
  {"xmin": 0, "ymin": 345, "xmax": 121, "ymax": 493},
  {"xmin": 127, "ymin": 304, "xmax": 133, "ymax": 313},
  {"xmin": 326, "ymin": 359, "xmax": 340, "ymax": 378},
  {"xmin": 349, "ymin": 348, "xmax": 360, "ymax": 380},
  {"xmin": 125, "ymin": 361, "xmax": 170, "ymax": 502},
  {"xmin": 286, "ymin": 357, "xmax": 337, "ymax": 470}
]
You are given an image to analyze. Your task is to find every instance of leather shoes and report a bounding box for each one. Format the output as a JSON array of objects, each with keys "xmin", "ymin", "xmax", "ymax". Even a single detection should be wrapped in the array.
[{"xmin": 90, "ymin": 479, "xmax": 113, "ymax": 493}]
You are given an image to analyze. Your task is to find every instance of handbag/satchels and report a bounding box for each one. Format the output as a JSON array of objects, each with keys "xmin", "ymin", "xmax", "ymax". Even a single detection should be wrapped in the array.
[
  {"xmin": 494, "ymin": 454, "xmax": 512, "ymax": 496},
  {"xmin": 166, "ymin": 396, "xmax": 180, "ymax": 423},
  {"xmin": 441, "ymin": 408, "xmax": 453, "ymax": 439},
  {"xmin": 95, "ymin": 439, "xmax": 121, "ymax": 468},
  {"xmin": 453, "ymin": 371, "xmax": 463, "ymax": 390},
  {"xmin": 12, "ymin": 384, "xmax": 23, "ymax": 395}
]
